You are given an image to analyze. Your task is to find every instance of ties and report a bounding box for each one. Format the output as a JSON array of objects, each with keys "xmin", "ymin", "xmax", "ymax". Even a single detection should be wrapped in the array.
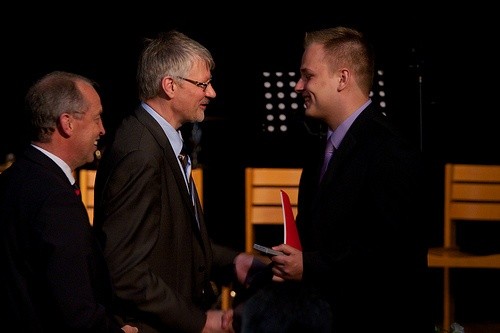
[
  {"xmin": 177, "ymin": 150, "xmax": 191, "ymax": 191},
  {"xmin": 320, "ymin": 135, "xmax": 337, "ymax": 183},
  {"xmin": 70, "ymin": 181, "xmax": 81, "ymax": 197}
]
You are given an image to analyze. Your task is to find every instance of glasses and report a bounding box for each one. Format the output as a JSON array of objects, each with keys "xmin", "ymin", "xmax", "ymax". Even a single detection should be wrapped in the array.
[{"xmin": 167, "ymin": 75, "xmax": 213, "ymax": 93}]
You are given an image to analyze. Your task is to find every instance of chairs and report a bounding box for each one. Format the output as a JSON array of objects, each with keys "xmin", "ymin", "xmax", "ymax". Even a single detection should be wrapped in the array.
[
  {"xmin": 79, "ymin": 168, "xmax": 204, "ymax": 227},
  {"xmin": 427, "ymin": 164, "xmax": 500, "ymax": 333},
  {"xmin": 222, "ymin": 168, "xmax": 303, "ymax": 310}
]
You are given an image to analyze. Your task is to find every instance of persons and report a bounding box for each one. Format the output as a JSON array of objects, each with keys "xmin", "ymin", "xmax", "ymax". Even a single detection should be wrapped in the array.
[
  {"xmin": 91, "ymin": 32, "xmax": 256, "ymax": 333},
  {"xmin": 0, "ymin": 72, "xmax": 140, "ymax": 333},
  {"xmin": 221, "ymin": 27, "xmax": 443, "ymax": 333}
]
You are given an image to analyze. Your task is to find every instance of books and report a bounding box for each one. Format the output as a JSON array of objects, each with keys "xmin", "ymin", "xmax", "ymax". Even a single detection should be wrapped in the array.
[{"xmin": 280, "ymin": 189, "xmax": 302, "ymax": 251}]
